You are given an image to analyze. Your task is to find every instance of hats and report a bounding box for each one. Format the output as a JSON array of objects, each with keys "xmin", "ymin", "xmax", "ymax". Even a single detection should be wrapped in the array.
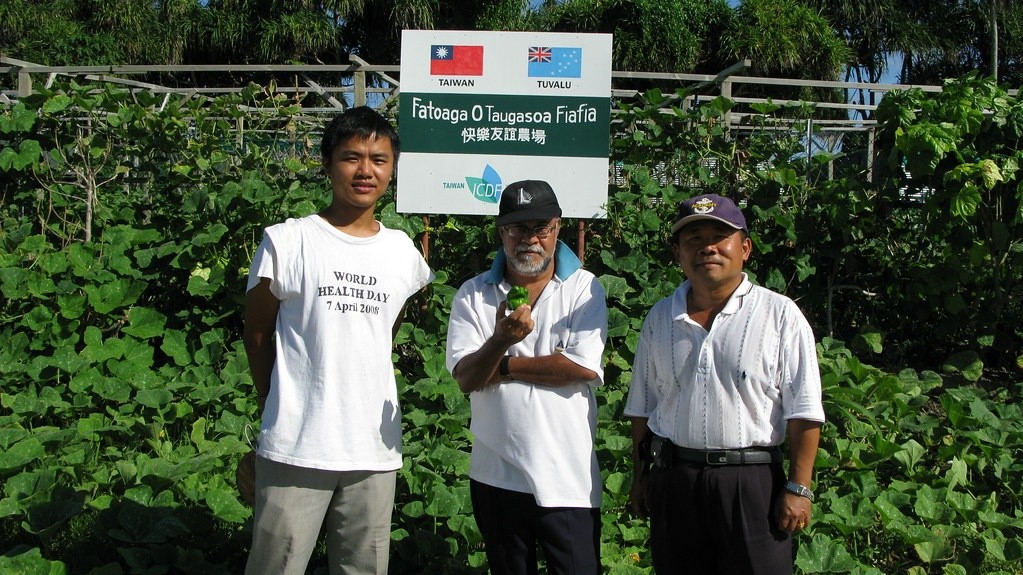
[
  {"xmin": 498, "ymin": 180, "xmax": 562, "ymax": 225},
  {"xmin": 670, "ymin": 194, "xmax": 748, "ymax": 236}
]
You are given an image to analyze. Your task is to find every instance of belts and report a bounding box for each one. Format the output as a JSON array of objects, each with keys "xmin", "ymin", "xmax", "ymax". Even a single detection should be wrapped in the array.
[{"xmin": 674, "ymin": 446, "xmax": 779, "ymax": 465}]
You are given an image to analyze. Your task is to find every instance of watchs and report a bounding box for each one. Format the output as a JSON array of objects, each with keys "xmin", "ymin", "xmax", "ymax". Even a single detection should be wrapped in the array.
[
  {"xmin": 500, "ymin": 355, "xmax": 512, "ymax": 382},
  {"xmin": 786, "ymin": 481, "xmax": 813, "ymax": 499}
]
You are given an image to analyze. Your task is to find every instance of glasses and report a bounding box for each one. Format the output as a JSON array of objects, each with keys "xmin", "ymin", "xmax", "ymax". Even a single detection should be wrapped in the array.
[{"xmin": 503, "ymin": 222, "xmax": 557, "ymax": 238}]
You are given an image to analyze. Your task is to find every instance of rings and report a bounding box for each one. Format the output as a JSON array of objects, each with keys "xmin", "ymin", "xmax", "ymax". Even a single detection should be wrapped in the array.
[{"xmin": 798, "ymin": 523, "xmax": 805, "ymax": 529}]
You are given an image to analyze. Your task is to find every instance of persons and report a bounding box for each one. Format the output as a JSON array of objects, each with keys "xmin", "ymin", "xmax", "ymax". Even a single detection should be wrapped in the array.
[
  {"xmin": 446, "ymin": 180, "xmax": 607, "ymax": 575},
  {"xmin": 243, "ymin": 106, "xmax": 436, "ymax": 575},
  {"xmin": 622, "ymin": 194, "xmax": 825, "ymax": 575}
]
